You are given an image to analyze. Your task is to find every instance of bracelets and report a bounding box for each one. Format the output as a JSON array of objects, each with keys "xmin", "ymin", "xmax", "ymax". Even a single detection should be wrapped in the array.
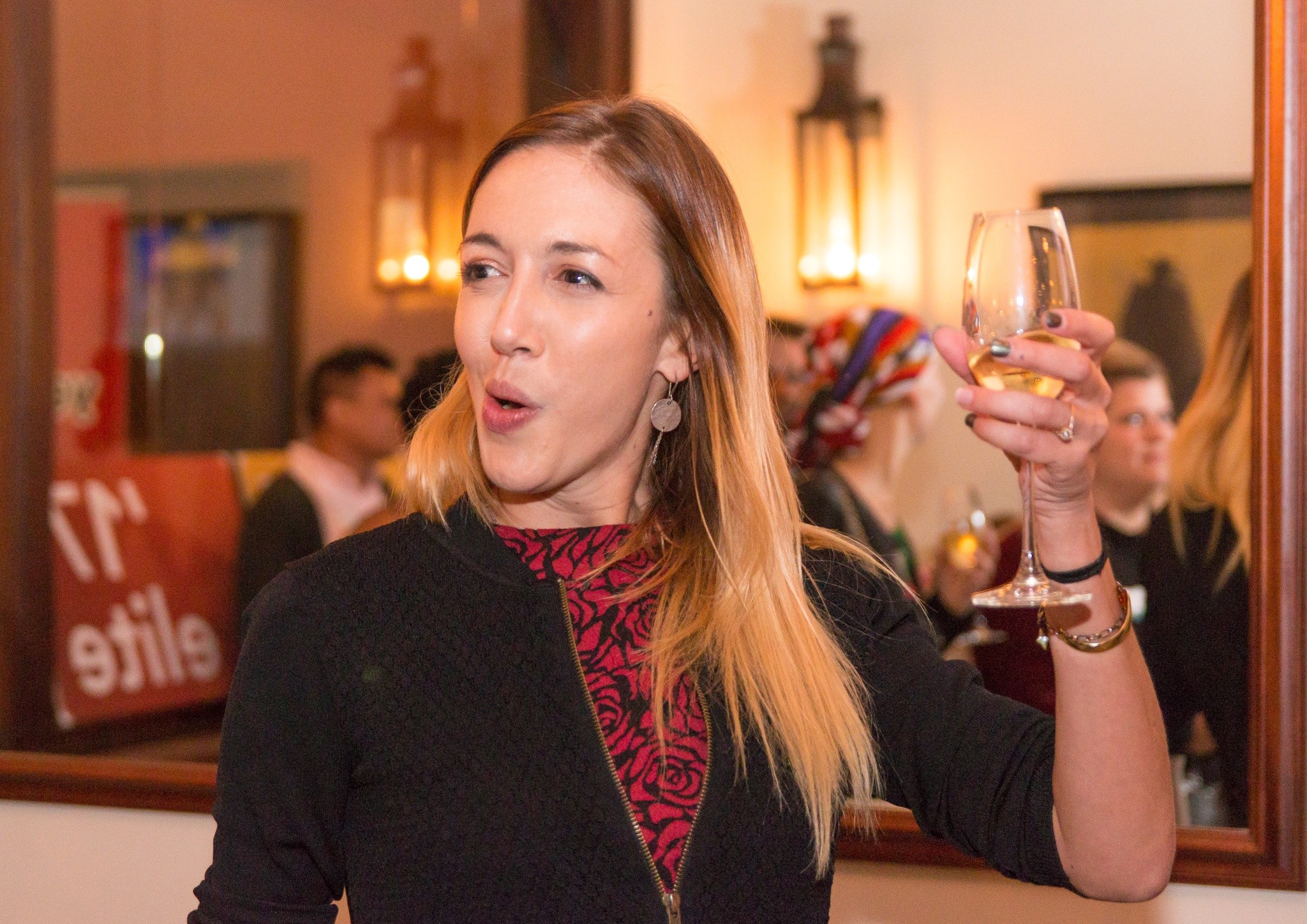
[
  {"xmin": 1040, "ymin": 536, "xmax": 1108, "ymax": 583},
  {"xmin": 1036, "ymin": 582, "xmax": 1132, "ymax": 653}
]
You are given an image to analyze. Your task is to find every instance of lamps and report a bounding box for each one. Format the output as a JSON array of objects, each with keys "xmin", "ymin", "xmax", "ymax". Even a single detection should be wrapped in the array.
[
  {"xmin": 371, "ymin": 43, "xmax": 461, "ymax": 293},
  {"xmin": 787, "ymin": 18, "xmax": 896, "ymax": 289}
]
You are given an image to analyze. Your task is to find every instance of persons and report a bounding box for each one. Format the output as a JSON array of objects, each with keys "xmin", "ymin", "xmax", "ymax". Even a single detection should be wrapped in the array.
[
  {"xmin": 233, "ymin": 314, "xmax": 906, "ymax": 664},
  {"xmin": 963, "ymin": 341, "xmax": 1250, "ymax": 831},
  {"xmin": 193, "ymin": 97, "xmax": 1177, "ymax": 924}
]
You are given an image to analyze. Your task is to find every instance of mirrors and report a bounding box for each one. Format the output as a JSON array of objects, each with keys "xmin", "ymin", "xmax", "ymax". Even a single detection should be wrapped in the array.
[{"xmin": 1, "ymin": 1, "xmax": 1306, "ymax": 894}]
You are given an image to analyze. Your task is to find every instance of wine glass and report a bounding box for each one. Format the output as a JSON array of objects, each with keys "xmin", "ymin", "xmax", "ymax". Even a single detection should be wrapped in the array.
[{"xmin": 960, "ymin": 206, "xmax": 1093, "ymax": 609}]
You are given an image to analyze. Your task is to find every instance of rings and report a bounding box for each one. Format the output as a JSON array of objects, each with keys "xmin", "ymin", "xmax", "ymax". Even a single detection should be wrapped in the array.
[{"xmin": 1055, "ymin": 403, "xmax": 1075, "ymax": 442}]
